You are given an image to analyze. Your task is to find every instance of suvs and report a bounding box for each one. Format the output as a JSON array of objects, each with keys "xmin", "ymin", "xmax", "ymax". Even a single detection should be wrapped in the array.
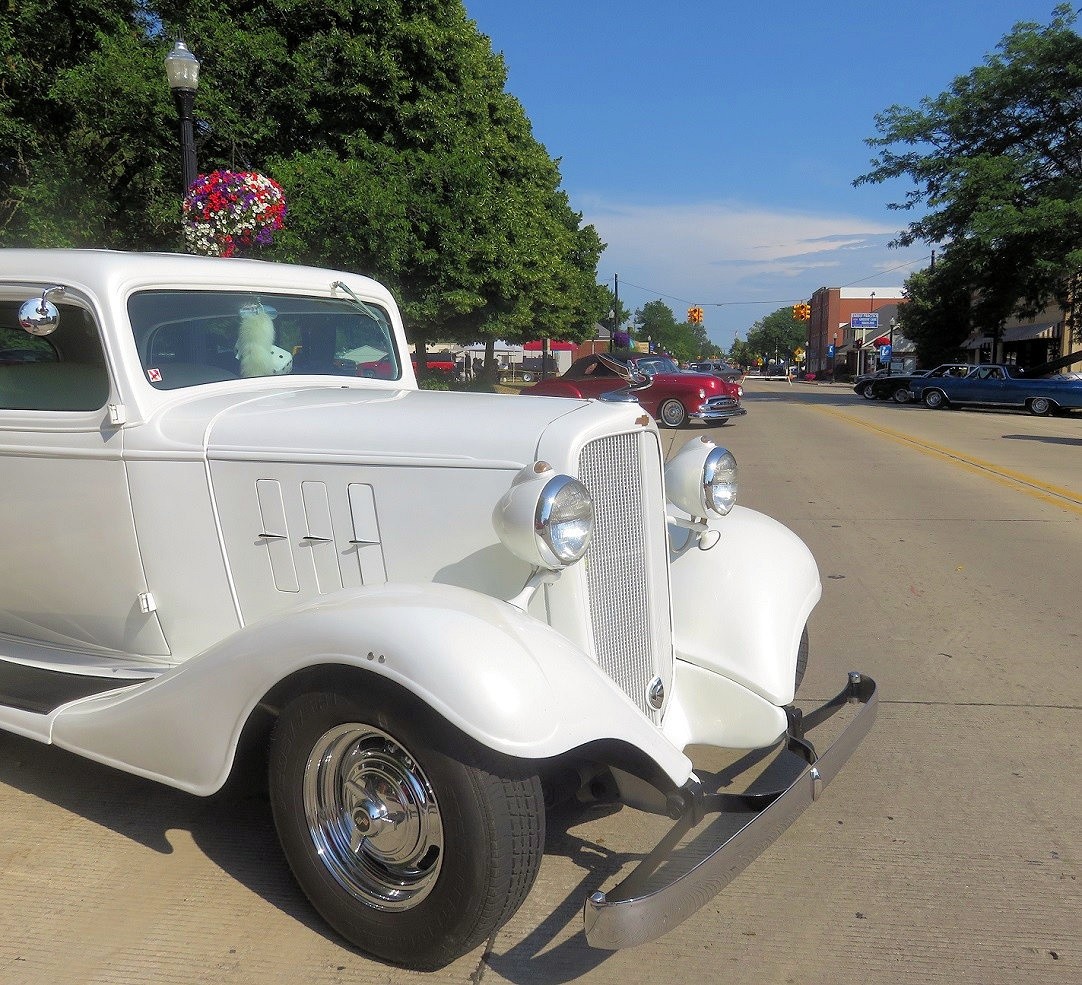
[{"xmin": 356, "ymin": 352, "xmax": 460, "ymax": 385}]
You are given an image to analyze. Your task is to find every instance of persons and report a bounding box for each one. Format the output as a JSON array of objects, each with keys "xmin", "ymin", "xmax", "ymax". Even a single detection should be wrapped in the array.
[{"xmin": 452, "ymin": 351, "xmax": 500, "ymax": 385}]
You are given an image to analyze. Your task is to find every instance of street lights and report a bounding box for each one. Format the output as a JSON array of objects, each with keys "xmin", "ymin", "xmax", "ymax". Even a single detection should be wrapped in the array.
[
  {"xmin": 647, "ymin": 335, "xmax": 652, "ymax": 354},
  {"xmin": 833, "ymin": 332, "xmax": 838, "ymax": 383},
  {"xmin": 608, "ymin": 308, "xmax": 615, "ymax": 352},
  {"xmin": 804, "ymin": 340, "xmax": 810, "ymax": 374},
  {"xmin": 887, "ymin": 315, "xmax": 897, "ymax": 377},
  {"xmin": 163, "ymin": 41, "xmax": 201, "ymax": 199}
]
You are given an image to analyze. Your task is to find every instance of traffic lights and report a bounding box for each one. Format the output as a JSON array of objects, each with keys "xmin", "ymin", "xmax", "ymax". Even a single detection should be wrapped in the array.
[
  {"xmin": 791, "ymin": 304, "xmax": 811, "ymax": 321},
  {"xmin": 687, "ymin": 307, "xmax": 703, "ymax": 323}
]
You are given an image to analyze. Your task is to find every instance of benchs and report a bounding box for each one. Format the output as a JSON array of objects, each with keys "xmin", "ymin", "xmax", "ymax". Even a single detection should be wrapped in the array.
[{"xmin": 0, "ymin": 358, "xmax": 235, "ymax": 411}]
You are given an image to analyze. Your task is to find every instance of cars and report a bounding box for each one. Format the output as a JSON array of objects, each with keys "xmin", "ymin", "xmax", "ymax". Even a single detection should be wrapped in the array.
[
  {"xmin": 688, "ymin": 362, "xmax": 742, "ymax": 383},
  {"xmin": 518, "ymin": 351, "xmax": 747, "ymax": 429},
  {"xmin": 765, "ymin": 364, "xmax": 787, "ymax": 380},
  {"xmin": 748, "ymin": 367, "xmax": 761, "ymax": 375},
  {"xmin": 848, "ymin": 363, "xmax": 1082, "ymax": 416},
  {"xmin": 0, "ymin": 246, "xmax": 880, "ymax": 973}
]
